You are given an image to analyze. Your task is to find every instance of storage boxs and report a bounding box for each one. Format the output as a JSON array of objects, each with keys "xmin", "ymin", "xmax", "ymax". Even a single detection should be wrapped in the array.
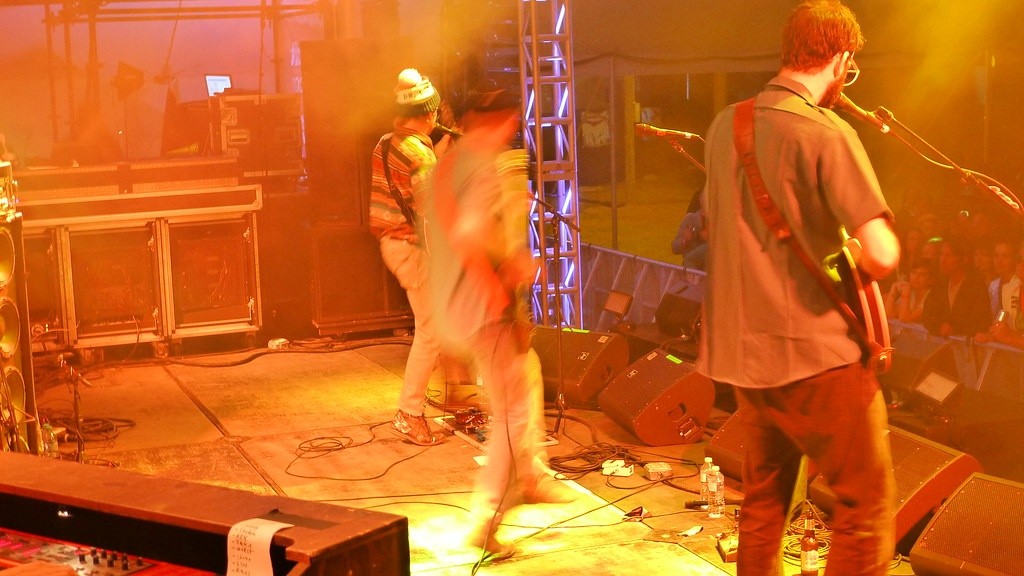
[{"xmin": 209, "ymin": 93, "xmax": 305, "ymax": 179}]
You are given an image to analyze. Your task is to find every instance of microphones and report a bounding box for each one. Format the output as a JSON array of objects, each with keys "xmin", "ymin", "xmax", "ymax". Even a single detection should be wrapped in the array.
[
  {"xmin": 435, "ymin": 123, "xmax": 463, "ymax": 137},
  {"xmin": 834, "ymin": 92, "xmax": 895, "ymax": 136},
  {"xmin": 635, "ymin": 124, "xmax": 700, "ymax": 139}
]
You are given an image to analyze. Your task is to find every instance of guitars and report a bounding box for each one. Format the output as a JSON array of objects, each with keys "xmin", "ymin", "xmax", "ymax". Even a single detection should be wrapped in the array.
[
  {"xmin": 842, "ymin": 225, "xmax": 893, "ymax": 373},
  {"xmin": 421, "ymin": 99, "xmax": 459, "ymax": 258}
]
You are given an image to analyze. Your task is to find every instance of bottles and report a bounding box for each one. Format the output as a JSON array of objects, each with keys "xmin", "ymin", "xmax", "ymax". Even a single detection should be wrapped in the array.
[
  {"xmin": 707, "ymin": 466, "xmax": 725, "ymax": 518},
  {"xmin": 699, "ymin": 456, "xmax": 716, "ymax": 509},
  {"xmin": 801, "ymin": 511, "xmax": 818, "ymax": 576}
]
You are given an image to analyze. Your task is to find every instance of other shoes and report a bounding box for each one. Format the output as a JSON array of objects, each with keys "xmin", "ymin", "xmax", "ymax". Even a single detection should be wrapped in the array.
[
  {"xmin": 446, "ymin": 391, "xmax": 488, "ymax": 412},
  {"xmin": 389, "ymin": 410, "xmax": 445, "ymax": 446}
]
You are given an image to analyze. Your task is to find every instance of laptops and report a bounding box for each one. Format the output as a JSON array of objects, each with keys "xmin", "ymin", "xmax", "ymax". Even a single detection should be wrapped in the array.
[{"xmin": 204, "ymin": 74, "xmax": 233, "ymax": 98}]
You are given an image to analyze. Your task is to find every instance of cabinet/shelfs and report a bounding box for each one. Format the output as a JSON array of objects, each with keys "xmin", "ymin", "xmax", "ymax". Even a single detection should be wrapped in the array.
[{"xmin": 12, "ymin": 184, "xmax": 265, "ymax": 352}]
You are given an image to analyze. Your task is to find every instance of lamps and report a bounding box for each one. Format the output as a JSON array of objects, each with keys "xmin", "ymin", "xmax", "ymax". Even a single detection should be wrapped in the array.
[
  {"xmin": 603, "ymin": 290, "xmax": 633, "ymax": 323},
  {"xmin": 913, "ymin": 366, "xmax": 963, "ymax": 408}
]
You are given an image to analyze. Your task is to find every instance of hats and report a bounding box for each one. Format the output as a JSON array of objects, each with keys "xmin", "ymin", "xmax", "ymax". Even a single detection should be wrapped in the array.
[{"xmin": 394, "ymin": 68, "xmax": 441, "ymax": 117}]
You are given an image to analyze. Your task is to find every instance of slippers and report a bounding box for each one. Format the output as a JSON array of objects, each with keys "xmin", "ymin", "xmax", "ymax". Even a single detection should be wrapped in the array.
[{"xmin": 481, "ymin": 543, "xmax": 516, "ymax": 566}]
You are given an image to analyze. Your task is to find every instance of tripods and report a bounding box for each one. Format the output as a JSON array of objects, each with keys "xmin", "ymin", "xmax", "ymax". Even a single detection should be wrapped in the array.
[
  {"xmin": 527, "ymin": 190, "xmax": 603, "ymax": 438},
  {"xmin": 55, "ymin": 358, "xmax": 119, "ymax": 469}
]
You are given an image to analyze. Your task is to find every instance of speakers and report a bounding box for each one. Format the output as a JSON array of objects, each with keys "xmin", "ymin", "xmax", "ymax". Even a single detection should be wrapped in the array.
[
  {"xmin": 596, "ymin": 348, "xmax": 715, "ymax": 447},
  {"xmin": 909, "ymin": 472, "xmax": 1024, "ymax": 576},
  {"xmin": 966, "ymin": 349, "xmax": 1024, "ymax": 411},
  {"xmin": 655, "ymin": 292, "xmax": 702, "ymax": 344},
  {"xmin": 808, "ymin": 423, "xmax": 985, "ymax": 558},
  {"xmin": 887, "ymin": 336, "xmax": 960, "ymax": 404},
  {"xmin": 704, "ymin": 406, "xmax": 745, "ymax": 484},
  {"xmin": 527, "ymin": 326, "xmax": 630, "ymax": 410}
]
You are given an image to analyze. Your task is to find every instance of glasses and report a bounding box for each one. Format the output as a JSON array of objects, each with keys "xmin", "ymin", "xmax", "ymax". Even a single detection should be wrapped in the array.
[{"xmin": 843, "ymin": 59, "xmax": 860, "ymax": 87}]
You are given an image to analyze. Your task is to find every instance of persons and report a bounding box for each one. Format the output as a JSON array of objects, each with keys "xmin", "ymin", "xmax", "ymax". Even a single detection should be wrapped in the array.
[
  {"xmin": 425, "ymin": 80, "xmax": 578, "ymax": 560},
  {"xmin": 369, "ymin": 68, "xmax": 485, "ymax": 447},
  {"xmin": 885, "ymin": 172, "xmax": 1024, "ymax": 349},
  {"xmin": 671, "ymin": 185, "xmax": 708, "ymax": 272},
  {"xmin": 696, "ymin": 0, "xmax": 901, "ymax": 576}
]
